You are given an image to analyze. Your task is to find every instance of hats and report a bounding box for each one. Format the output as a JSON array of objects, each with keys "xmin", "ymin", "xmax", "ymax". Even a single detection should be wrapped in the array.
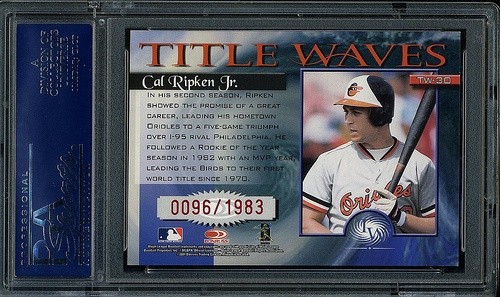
[{"xmin": 333, "ymin": 75, "xmax": 394, "ymax": 110}]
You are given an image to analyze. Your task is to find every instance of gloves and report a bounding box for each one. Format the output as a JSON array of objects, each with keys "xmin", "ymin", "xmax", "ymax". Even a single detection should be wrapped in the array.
[{"xmin": 373, "ymin": 186, "xmax": 406, "ymax": 227}]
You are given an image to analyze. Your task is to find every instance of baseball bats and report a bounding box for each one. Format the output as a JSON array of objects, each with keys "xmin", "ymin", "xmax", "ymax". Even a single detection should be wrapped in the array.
[{"xmin": 386, "ymin": 88, "xmax": 436, "ymax": 193}]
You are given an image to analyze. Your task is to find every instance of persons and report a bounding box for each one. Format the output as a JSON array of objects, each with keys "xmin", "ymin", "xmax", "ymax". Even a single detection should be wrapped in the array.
[{"xmin": 302, "ymin": 74, "xmax": 436, "ymax": 235}]
